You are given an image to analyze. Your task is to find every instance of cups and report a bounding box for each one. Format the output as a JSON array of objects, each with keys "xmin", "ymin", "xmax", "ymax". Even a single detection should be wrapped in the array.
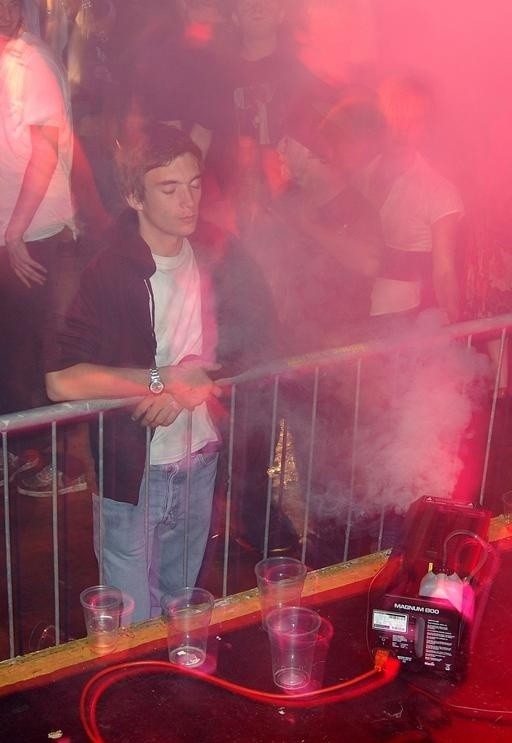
[
  {"xmin": 159, "ymin": 588, "xmax": 214, "ymax": 668},
  {"xmin": 254, "ymin": 554, "xmax": 305, "ymax": 633},
  {"xmin": 265, "ymin": 605, "xmax": 319, "ymax": 690},
  {"xmin": 79, "ymin": 584, "xmax": 123, "ymax": 654}
]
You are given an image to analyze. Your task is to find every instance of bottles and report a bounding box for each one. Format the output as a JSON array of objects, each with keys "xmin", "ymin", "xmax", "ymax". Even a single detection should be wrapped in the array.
[
  {"xmin": 277, "ymin": 611, "xmax": 333, "ymax": 698},
  {"xmin": 420, "ymin": 568, "xmax": 476, "ymax": 620}
]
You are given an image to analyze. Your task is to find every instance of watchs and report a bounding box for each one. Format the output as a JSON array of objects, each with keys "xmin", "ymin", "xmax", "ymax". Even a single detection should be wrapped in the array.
[{"xmin": 145, "ymin": 365, "xmax": 166, "ymax": 393}]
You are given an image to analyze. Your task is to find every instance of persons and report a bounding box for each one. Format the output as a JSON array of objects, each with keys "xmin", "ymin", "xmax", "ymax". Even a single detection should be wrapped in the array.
[
  {"xmin": 37, "ymin": 123, "xmax": 276, "ymax": 634},
  {"xmin": 0, "ymin": 3, "xmax": 509, "ymax": 558}
]
